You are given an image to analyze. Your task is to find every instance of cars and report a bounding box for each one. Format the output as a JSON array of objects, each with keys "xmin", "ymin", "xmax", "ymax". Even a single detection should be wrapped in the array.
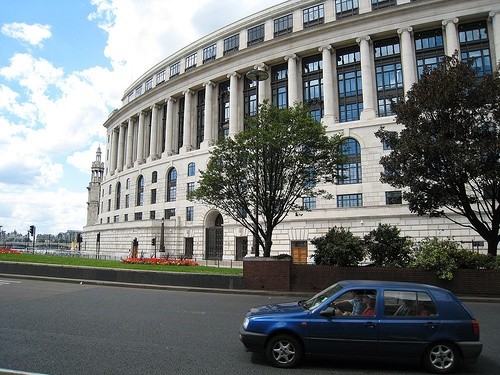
[{"xmin": 238, "ymin": 280, "xmax": 483, "ymax": 374}]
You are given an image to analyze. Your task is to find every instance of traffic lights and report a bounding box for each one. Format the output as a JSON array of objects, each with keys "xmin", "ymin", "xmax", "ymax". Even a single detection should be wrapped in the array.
[
  {"xmin": 151, "ymin": 238, "xmax": 155, "ymax": 246},
  {"xmin": 80, "ymin": 237, "xmax": 84, "ymax": 243},
  {"xmin": 29, "ymin": 225, "xmax": 34, "ymax": 235},
  {"xmin": 133, "ymin": 239, "xmax": 137, "ymax": 247}
]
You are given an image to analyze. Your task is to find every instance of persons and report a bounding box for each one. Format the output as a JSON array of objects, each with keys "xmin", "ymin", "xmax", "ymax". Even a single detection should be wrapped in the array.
[
  {"xmin": 127, "ymin": 249, "xmax": 131, "ymax": 259},
  {"xmin": 334, "ymin": 289, "xmax": 377, "ymax": 316},
  {"xmin": 391, "ymin": 292, "xmax": 417, "ymax": 317}
]
